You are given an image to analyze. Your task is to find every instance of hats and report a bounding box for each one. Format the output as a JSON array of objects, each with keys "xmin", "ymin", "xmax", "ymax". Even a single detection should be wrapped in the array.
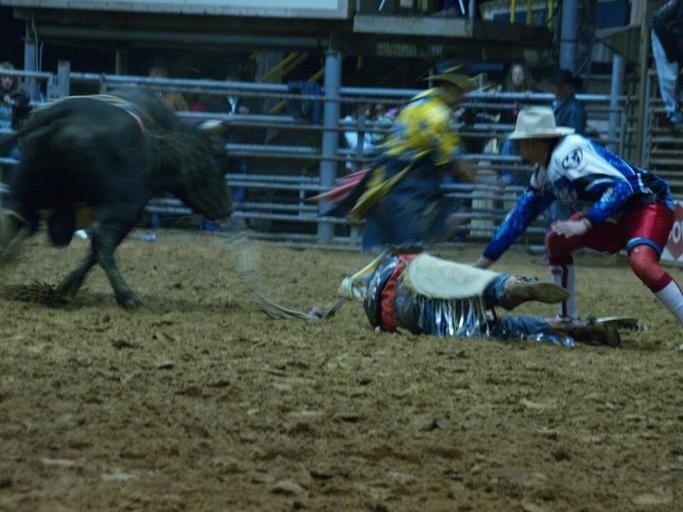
[
  {"xmin": 503, "ymin": 104, "xmax": 577, "ymax": 142},
  {"xmin": 424, "ymin": 54, "xmax": 478, "ymax": 92}
]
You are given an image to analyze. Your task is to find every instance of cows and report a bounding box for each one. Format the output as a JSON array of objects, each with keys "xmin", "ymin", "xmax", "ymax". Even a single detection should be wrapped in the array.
[{"xmin": 0, "ymin": 83, "xmax": 234, "ymax": 310}]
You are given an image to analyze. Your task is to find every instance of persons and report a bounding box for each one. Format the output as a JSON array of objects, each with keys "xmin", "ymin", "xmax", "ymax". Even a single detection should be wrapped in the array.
[
  {"xmin": 467, "ymin": 106, "xmax": 683, "ymax": 325},
  {"xmin": 362, "ymin": 240, "xmax": 622, "ymax": 348},
  {"xmin": 147, "ymin": 62, "xmax": 189, "ymax": 115},
  {"xmin": 200, "ymin": 72, "xmax": 249, "ymax": 232},
  {"xmin": 0, "ymin": 62, "xmax": 22, "ymax": 196}
]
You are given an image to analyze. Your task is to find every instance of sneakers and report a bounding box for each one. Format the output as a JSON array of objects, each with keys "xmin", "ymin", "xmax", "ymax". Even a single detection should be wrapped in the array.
[
  {"xmin": 575, "ymin": 319, "xmax": 624, "ymax": 349},
  {"xmin": 504, "ymin": 278, "xmax": 574, "ymax": 304}
]
